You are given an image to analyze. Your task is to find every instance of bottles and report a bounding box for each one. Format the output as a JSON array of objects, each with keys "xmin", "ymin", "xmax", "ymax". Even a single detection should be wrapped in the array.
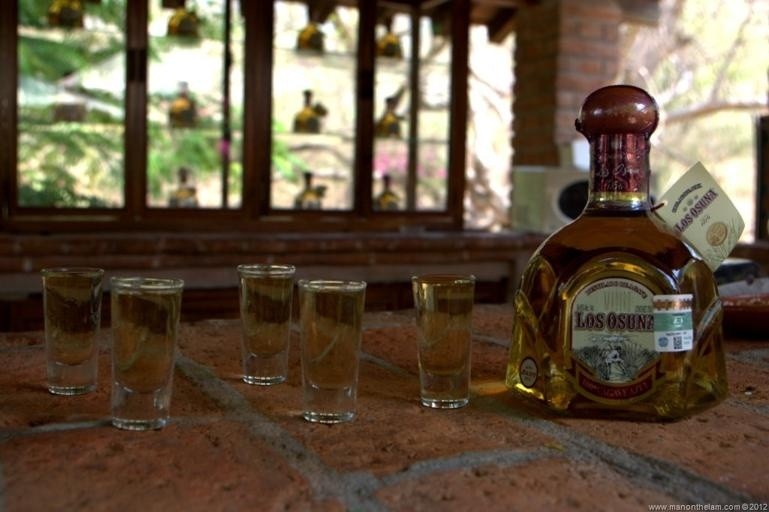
[
  {"xmin": 294, "ymin": 174, "xmax": 322, "ymax": 211},
  {"xmin": 167, "ymin": 168, "xmax": 198, "ymax": 207},
  {"xmin": 292, "ymin": 90, "xmax": 322, "ymax": 131},
  {"xmin": 167, "ymin": 1, "xmax": 199, "ymax": 36},
  {"xmin": 375, "ymin": 176, "xmax": 399, "ymax": 211},
  {"xmin": 375, "ymin": 99, "xmax": 401, "ymax": 136},
  {"xmin": 296, "ymin": 10, "xmax": 325, "ymax": 50},
  {"xmin": 168, "ymin": 82, "xmax": 196, "ymax": 126},
  {"xmin": 54, "ymin": 73, "xmax": 86, "ymax": 122},
  {"xmin": 379, "ymin": 19, "xmax": 403, "ymax": 58},
  {"xmin": 48, "ymin": 1, "xmax": 83, "ymax": 30},
  {"xmin": 506, "ymin": 86, "xmax": 729, "ymax": 419}
]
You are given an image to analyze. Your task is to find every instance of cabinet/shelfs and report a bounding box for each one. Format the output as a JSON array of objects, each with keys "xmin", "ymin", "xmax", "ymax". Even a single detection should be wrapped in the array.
[{"xmin": 1, "ymin": 0, "xmax": 470, "ymax": 234}]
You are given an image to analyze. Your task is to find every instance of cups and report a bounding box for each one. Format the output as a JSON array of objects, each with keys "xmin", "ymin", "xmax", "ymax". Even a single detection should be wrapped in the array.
[
  {"xmin": 108, "ymin": 276, "xmax": 186, "ymax": 431},
  {"xmin": 297, "ymin": 279, "xmax": 366, "ymax": 425},
  {"xmin": 410, "ymin": 273, "xmax": 477, "ymax": 410},
  {"xmin": 237, "ymin": 262, "xmax": 295, "ymax": 387},
  {"xmin": 40, "ymin": 265, "xmax": 104, "ymax": 395}
]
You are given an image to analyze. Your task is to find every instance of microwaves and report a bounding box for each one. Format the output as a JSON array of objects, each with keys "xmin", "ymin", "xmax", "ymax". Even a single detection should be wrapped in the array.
[{"xmin": 510, "ymin": 165, "xmax": 590, "ymax": 234}]
